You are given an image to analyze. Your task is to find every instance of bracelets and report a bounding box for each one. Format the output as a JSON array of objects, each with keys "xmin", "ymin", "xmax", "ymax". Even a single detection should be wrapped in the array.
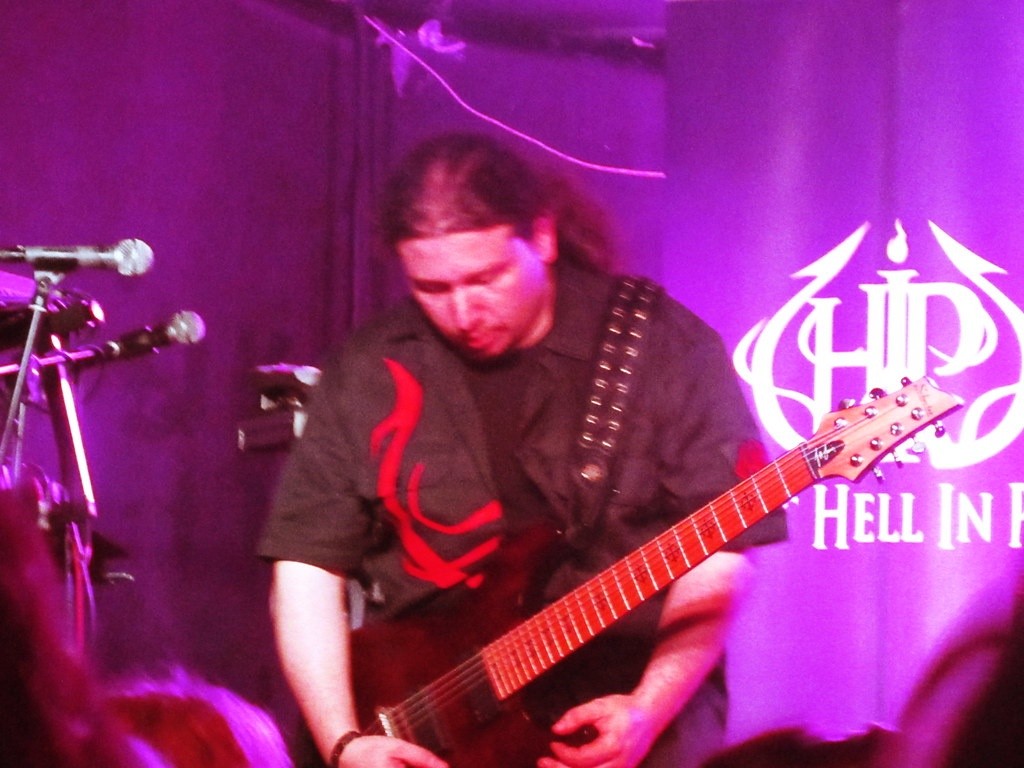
[{"xmin": 329, "ymin": 731, "xmax": 362, "ymax": 768}]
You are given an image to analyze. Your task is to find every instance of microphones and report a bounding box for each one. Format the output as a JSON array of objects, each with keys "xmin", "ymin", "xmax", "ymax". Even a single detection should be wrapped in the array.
[
  {"xmin": 105, "ymin": 311, "xmax": 205, "ymax": 357},
  {"xmin": 0, "ymin": 238, "xmax": 153, "ymax": 277}
]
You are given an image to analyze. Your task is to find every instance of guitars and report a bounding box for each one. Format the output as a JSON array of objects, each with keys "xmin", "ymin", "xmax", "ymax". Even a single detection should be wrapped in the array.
[{"xmin": 296, "ymin": 377, "xmax": 966, "ymax": 768}]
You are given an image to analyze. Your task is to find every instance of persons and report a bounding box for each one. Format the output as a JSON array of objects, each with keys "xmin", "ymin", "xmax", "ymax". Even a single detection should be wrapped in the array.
[{"xmin": 253, "ymin": 132, "xmax": 788, "ymax": 768}]
